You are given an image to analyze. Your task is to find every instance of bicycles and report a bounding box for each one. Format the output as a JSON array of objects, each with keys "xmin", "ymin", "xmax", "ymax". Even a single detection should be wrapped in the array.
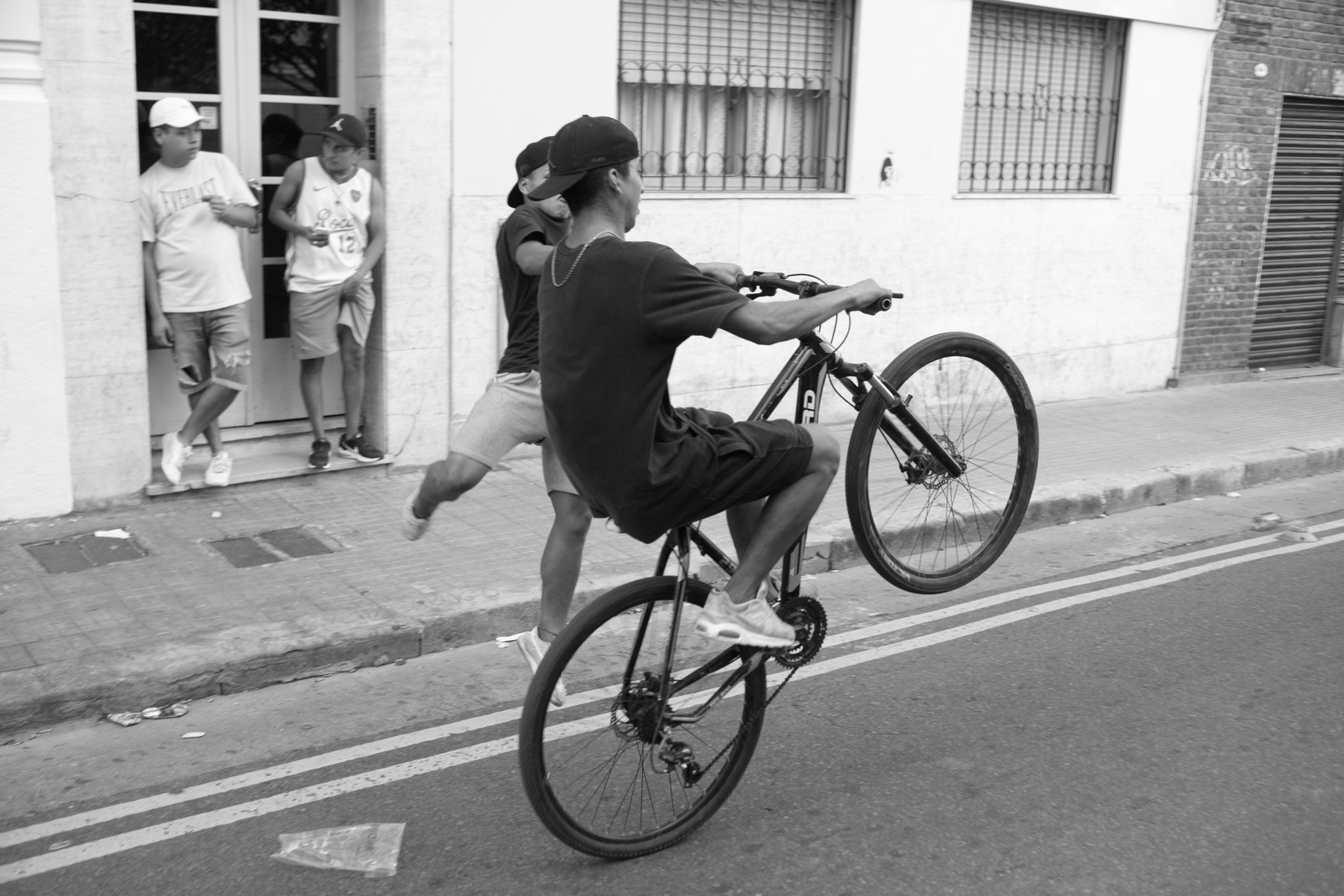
[{"xmin": 517, "ymin": 274, "xmax": 1042, "ymax": 859}]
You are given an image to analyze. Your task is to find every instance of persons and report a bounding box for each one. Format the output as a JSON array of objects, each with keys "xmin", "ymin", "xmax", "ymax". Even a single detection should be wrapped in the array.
[
  {"xmin": 525, "ymin": 114, "xmax": 893, "ymax": 647},
  {"xmin": 401, "ymin": 140, "xmax": 576, "ymax": 709},
  {"xmin": 268, "ymin": 113, "xmax": 386, "ymax": 466},
  {"xmin": 261, "ymin": 113, "xmax": 304, "ymax": 338},
  {"xmin": 140, "ymin": 96, "xmax": 260, "ymax": 486}
]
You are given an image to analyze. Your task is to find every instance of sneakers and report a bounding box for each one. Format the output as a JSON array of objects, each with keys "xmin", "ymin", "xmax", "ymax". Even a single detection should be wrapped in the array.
[
  {"xmin": 764, "ymin": 573, "xmax": 818, "ymax": 609},
  {"xmin": 517, "ymin": 626, "xmax": 566, "ymax": 707},
  {"xmin": 337, "ymin": 432, "xmax": 385, "ymax": 463},
  {"xmin": 307, "ymin": 439, "xmax": 332, "ymax": 469},
  {"xmin": 402, "ymin": 482, "xmax": 432, "ymax": 541},
  {"xmin": 205, "ymin": 451, "xmax": 232, "ymax": 486},
  {"xmin": 161, "ymin": 431, "xmax": 193, "ymax": 485},
  {"xmin": 693, "ymin": 580, "xmax": 795, "ymax": 648}
]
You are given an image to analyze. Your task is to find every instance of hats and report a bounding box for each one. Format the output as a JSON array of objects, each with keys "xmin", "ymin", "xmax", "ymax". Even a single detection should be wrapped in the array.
[
  {"xmin": 314, "ymin": 113, "xmax": 368, "ymax": 149},
  {"xmin": 526, "ymin": 115, "xmax": 639, "ymax": 201},
  {"xmin": 149, "ymin": 97, "xmax": 210, "ymax": 128},
  {"xmin": 507, "ymin": 135, "xmax": 555, "ymax": 208}
]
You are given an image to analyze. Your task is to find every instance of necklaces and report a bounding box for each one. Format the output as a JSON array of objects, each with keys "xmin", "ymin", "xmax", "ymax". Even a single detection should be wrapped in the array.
[{"xmin": 551, "ymin": 230, "xmax": 622, "ymax": 287}]
